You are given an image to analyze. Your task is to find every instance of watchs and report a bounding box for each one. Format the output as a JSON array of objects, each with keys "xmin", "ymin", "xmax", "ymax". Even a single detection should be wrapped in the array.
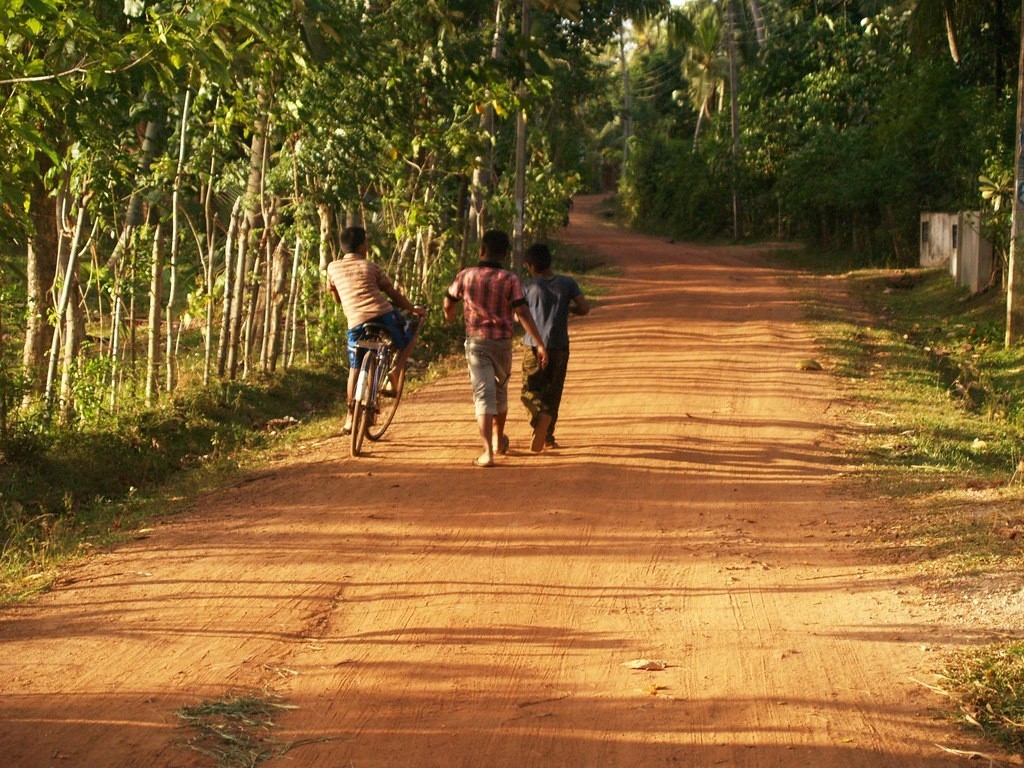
[{"xmin": 413, "ymin": 305, "xmax": 418, "ymax": 309}]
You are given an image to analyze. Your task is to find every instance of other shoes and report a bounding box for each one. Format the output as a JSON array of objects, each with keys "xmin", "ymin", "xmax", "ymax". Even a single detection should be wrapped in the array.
[
  {"xmin": 543, "ymin": 437, "xmax": 555, "ymax": 449},
  {"xmin": 530, "ymin": 414, "xmax": 552, "ymax": 452}
]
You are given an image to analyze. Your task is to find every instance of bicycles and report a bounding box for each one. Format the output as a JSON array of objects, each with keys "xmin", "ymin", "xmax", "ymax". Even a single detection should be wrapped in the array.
[{"xmin": 350, "ymin": 303, "xmax": 428, "ymax": 457}]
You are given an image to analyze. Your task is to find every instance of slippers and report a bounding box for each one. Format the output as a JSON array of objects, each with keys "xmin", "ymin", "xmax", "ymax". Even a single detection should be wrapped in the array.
[
  {"xmin": 473, "ymin": 458, "xmax": 492, "ymax": 467},
  {"xmin": 493, "ymin": 434, "xmax": 509, "ymax": 455}
]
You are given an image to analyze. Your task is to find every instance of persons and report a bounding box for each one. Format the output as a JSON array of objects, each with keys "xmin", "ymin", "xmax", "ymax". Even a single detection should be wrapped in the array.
[
  {"xmin": 562, "ymin": 194, "xmax": 573, "ymax": 210},
  {"xmin": 325, "ymin": 226, "xmax": 427, "ymax": 432},
  {"xmin": 518, "ymin": 241, "xmax": 590, "ymax": 451},
  {"xmin": 442, "ymin": 229, "xmax": 549, "ymax": 467}
]
